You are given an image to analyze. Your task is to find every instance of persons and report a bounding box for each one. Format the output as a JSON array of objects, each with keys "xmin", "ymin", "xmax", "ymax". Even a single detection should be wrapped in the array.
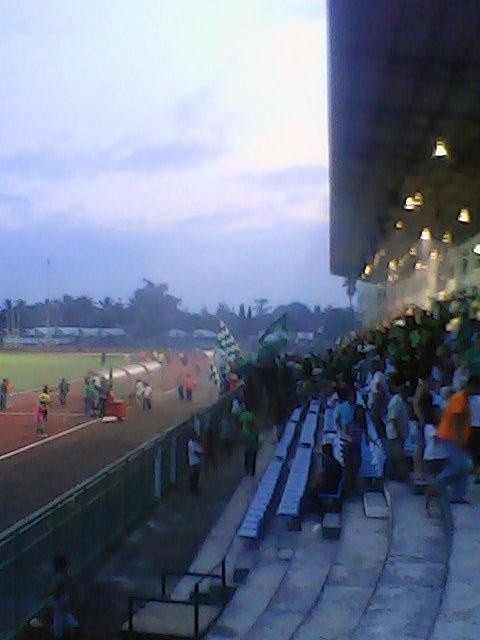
[
  {"xmin": 188, "ymin": 431, "xmax": 204, "ymax": 492},
  {"xmin": 0, "ymin": 376, "xmax": 14, "ymax": 413},
  {"xmin": 36, "ymin": 346, "xmax": 196, "ymax": 440}
]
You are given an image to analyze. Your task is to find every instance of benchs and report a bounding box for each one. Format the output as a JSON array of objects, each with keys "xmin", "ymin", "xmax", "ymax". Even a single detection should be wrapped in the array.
[{"xmin": 238, "ymin": 393, "xmax": 387, "ymax": 549}]
